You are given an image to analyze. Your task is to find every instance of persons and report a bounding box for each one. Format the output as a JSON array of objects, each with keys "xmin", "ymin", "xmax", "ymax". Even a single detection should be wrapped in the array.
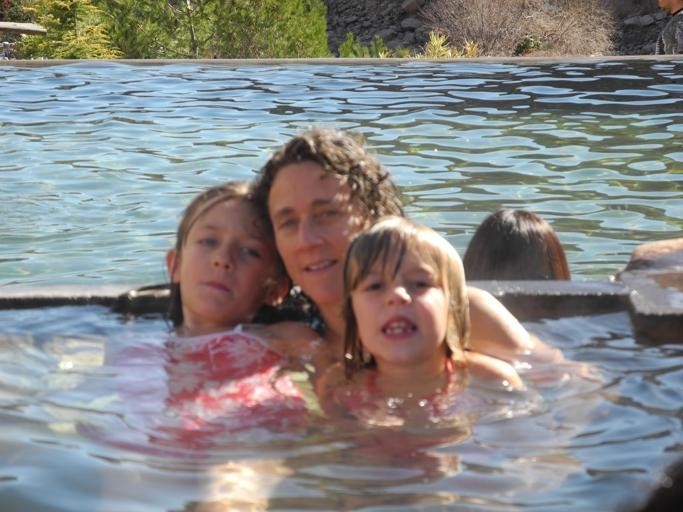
[
  {"xmin": 86, "ymin": 175, "xmax": 313, "ymax": 512},
  {"xmin": 313, "ymin": 215, "xmax": 525, "ymax": 412},
  {"xmin": 462, "ymin": 206, "xmax": 571, "ymax": 283},
  {"xmin": 261, "ymin": 118, "xmax": 565, "ymax": 386}
]
